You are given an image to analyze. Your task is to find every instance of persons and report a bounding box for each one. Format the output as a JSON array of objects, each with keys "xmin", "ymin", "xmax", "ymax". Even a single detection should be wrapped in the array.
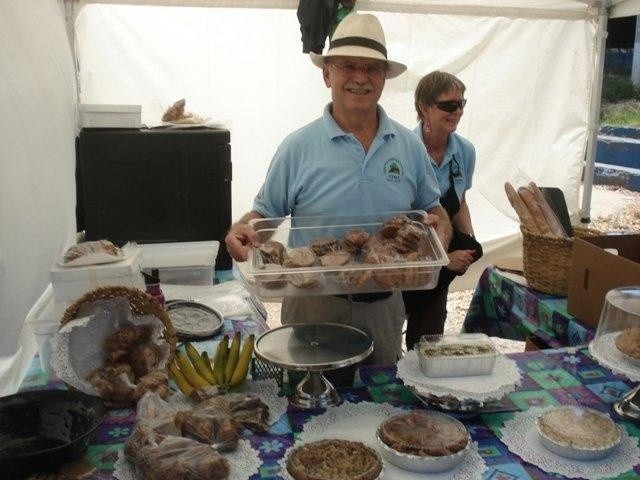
[
  {"xmin": 398, "ymin": 68, "xmax": 476, "ymax": 351},
  {"xmin": 221, "ymin": 12, "xmax": 454, "ymax": 390}
]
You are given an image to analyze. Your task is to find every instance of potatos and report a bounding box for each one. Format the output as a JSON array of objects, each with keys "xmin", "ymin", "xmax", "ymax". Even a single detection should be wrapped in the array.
[{"xmin": 85, "ymin": 324, "xmax": 266, "ymax": 480}]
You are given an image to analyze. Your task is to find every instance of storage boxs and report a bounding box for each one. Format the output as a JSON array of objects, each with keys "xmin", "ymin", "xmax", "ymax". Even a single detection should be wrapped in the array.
[
  {"xmin": 79, "ymin": 104, "xmax": 141, "ymax": 129},
  {"xmin": 566, "ymin": 233, "xmax": 640, "ymax": 329},
  {"xmin": 248, "ymin": 210, "xmax": 451, "ymax": 300},
  {"xmin": 23, "ymin": 240, "xmax": 220, "ymax": 375}
]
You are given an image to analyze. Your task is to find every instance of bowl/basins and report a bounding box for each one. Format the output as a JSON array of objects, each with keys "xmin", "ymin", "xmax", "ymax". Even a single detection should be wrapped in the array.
[{"xmin": 1, "ymin": 390, "xmax": 108, "ymax": 480}]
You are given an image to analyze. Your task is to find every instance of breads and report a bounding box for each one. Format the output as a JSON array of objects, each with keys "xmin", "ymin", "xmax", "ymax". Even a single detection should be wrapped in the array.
[
  {"xmin": 615, "ymin": 327, "xmax": 640, "ymax": 360},
  {"xmin": 63, "ymin": 239, "xmax": 119, "ymax": 265},
  {"xmin": 539, "ymin": 407, "xmax": 619, "ymax": 449},
  {"xmin": 503, "ymin": 180, "xmax": 569, "ymax": 238},
  {"xmin": 286, "ymin": 439, "xmax": 383, "ymax": 479},
  {"xmin": 378, "ymin": 416, "xmax": 467, "ymax": 455},
  {"xmin": 258, "ymin": 214, "xmax": 433, "ymax": 288}
]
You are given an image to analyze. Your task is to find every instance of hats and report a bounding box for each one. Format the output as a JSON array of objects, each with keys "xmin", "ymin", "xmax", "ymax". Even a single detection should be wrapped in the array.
[{"xmin": 309, "ymin": 13, "xmax": 407, "ymax": 79}]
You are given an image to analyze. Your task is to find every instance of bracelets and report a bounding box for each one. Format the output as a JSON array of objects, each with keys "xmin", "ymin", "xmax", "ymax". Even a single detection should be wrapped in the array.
[{"xmin": 468, "ymin": 232, "xmax": 476, "ymax": 239}]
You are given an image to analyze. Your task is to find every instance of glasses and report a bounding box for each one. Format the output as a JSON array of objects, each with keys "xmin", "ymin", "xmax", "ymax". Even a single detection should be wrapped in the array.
[
  {"xmin": 433, "ymin": 99, "xmax": 467, "ymax": 111},
  {"xmin": 329, "ymin": 61, "xmax": 384, "ymax": 77}
]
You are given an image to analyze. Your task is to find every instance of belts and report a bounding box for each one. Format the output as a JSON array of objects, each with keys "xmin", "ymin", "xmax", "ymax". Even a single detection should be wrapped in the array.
[{"xmin": 334, "ymin": 291, "xmax": 393, "ymax": 303}]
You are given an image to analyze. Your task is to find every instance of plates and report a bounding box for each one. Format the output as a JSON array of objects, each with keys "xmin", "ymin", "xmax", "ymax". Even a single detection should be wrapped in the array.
[
  {"xmin": 375, "ymin": 411, "xmax": 472, "ymax": 472},
  {"xmin": 536, "ymin": 407, "xmax": 624, "ymax": 460}
]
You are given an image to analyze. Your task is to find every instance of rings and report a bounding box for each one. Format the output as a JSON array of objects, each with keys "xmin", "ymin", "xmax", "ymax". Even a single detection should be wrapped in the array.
[{"xmin": 464, "ymin": 263, "xmax": 469, "ymax": 270}]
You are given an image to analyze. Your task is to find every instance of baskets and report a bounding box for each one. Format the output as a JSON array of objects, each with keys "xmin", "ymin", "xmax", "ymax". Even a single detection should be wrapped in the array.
[
  {"xmin": 60, "ymin": 285, "xmax": 177, "ymax": 409},
  {"xmin": 519, "ymin": 224, "xmax": 607, "ymax": 296}
]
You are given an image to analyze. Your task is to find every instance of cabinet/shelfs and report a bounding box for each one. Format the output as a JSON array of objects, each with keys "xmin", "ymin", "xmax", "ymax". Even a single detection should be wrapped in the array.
[{"xmin": 75, "ymin": 125, "xmax": 234, "ymax": 270}]
